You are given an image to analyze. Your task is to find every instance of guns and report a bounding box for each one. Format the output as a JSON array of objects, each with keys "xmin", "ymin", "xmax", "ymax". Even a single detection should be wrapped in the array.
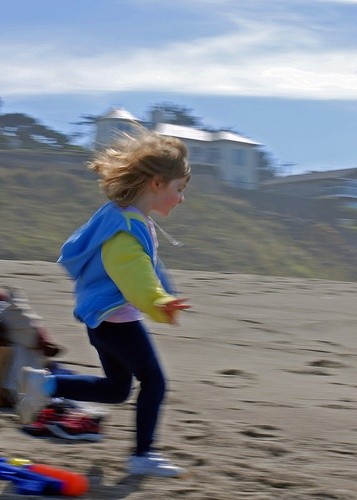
[{"xmin": 0, "ymin": 457, "xmax": 90, "ymax": 496}]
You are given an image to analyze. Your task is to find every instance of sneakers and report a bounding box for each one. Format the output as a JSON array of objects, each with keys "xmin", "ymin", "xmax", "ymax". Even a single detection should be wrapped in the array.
[
  {"xmin": 128, "ymin": 454, "xmax": 180, "ymax": 478},
  {"xmin": 19, "ymin": 365, "xmax": 51, "ymax": 425}
]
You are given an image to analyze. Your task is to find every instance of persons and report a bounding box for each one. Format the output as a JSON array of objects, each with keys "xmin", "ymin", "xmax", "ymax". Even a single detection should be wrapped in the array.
[{"xmin": 18, "ymin": 133, "xmax": 191, "ymax": 477}]
